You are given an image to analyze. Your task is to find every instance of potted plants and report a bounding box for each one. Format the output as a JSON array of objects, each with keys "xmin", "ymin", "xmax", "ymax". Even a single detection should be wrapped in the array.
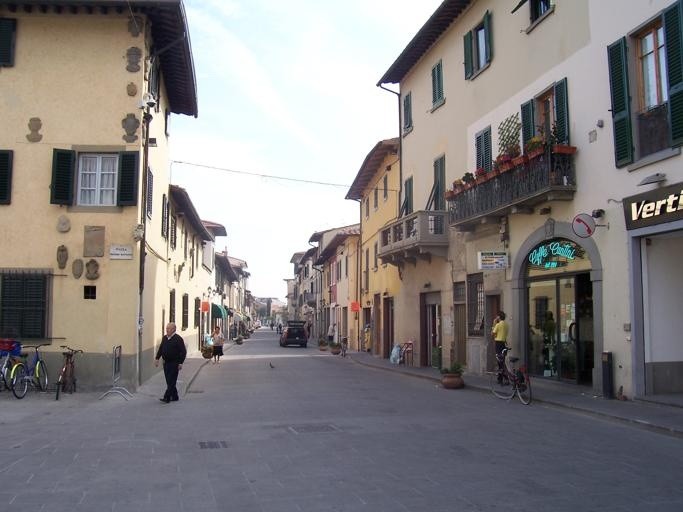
[
  {"xmin": 329, "ymin": 341, "xmax": 341, "ymax": 354},
  {"xmin": 439, "ymin": 358, "xmax": 466, "ymax": 389},
  {"xmin": 201, "ymin": 345, "xmax": 212, "ymax": 360},
  {"xmin": 236, "ymin": 336, "xmax": 243, "ymax": 345},
  {"xmin": 317, "ymin": 336, "xmax": 328, "ymax": 352}
]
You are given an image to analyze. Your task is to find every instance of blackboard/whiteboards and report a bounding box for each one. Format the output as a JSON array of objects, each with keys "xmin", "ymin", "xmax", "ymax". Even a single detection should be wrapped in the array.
[{"xmin": 0, "ymin": 267, "xmax": 53, "ymax": 345}]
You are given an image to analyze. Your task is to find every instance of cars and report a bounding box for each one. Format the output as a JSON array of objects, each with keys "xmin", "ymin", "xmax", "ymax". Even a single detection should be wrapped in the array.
[{"xmin": 255, "ymin": 319, "xmax": 270, "ymax": 329}]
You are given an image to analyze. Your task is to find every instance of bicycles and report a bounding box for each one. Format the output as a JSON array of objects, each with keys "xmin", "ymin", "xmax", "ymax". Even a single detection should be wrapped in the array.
[
  {"xmin": 341, "ymin": 336, "xmax": 352, "ymax": 358},
  {"xmin": 488, "ymin": 347, "xmax": 532, "ymax": 405},
  {"xmin": 0, "ymin": 336, "xmax": 86, "ymax": 401}
]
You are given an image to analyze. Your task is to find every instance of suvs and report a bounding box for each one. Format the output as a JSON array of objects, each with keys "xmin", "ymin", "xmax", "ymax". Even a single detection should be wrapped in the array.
[{"xmin": 279, "ymin": 320, "xmax": 307, "ymax": 348}]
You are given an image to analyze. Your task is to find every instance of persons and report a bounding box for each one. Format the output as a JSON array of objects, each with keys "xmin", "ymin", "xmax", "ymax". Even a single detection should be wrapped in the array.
[
  {"xmin": 208, "ymin": 325, "xmax": 225, "ymax": 365},
  {"xmin": 325, "ymin": 323, "xmax": 335, "ymax": 342},
  {"xmin": 154, "ymin": 321, "xmax": 187, "ymax": 403},
  {"xmin": 305, "ymin": 320, "xmax": 313, "ymax": 340},
  {"xmin": 540, "ymin": 310, "xmax": 555, "ymax": 365},
  {"xmin": 268, "ymin": 321, "xmax": 282, "ymax": 335},
  {"xmin": 490, "ymin": 311, "xmax": 511, "ymax": 387}
]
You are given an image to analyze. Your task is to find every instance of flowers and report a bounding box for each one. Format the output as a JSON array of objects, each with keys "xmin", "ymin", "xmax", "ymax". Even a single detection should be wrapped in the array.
[{"xmin": 448, "ymin": 135, "xmax": 551, "ymax": 191}]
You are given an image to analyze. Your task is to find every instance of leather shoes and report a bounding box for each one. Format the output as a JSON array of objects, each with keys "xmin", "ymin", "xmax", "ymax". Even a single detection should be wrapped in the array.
[
  {"xmin": 169, "ymin": 397, "xmax": 179, "ymax": 401},
  {"xmin": 159, "ymin": 398, "xmax": 169, "ymax": 403}
]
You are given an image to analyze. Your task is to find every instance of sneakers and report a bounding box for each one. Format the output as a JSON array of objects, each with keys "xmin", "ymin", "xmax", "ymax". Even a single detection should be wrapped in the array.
[
  {"xmin": 212, "ymin": 360, "xmax": 221, "ymax": 364},
  {"xmin": 496, "ymin": 380, "xmax": 510, "ymax": 386}
]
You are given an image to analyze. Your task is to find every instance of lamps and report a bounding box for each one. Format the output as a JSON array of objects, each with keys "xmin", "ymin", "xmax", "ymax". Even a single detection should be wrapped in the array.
[
  {"xmin": 210, "ymin": 288, "xmax": 216, "ymax": 298},
  {"xmin": 202, "ymin": 285, "xmax": 210, "ymax": 298},
  {"xmin": 536, "ymin": 205, "xmax": 552, "ymax": 217},
  {"xmin": 635, "ymin": 168, "xmax": 667, "ymax": 187},
  {"xmin": 591, "ymin": 208, "xmax": 606, "ymax": 218},
  {"xmin": 136, "ymin": 90, "xmax": 156, "ymax": 112}
]
